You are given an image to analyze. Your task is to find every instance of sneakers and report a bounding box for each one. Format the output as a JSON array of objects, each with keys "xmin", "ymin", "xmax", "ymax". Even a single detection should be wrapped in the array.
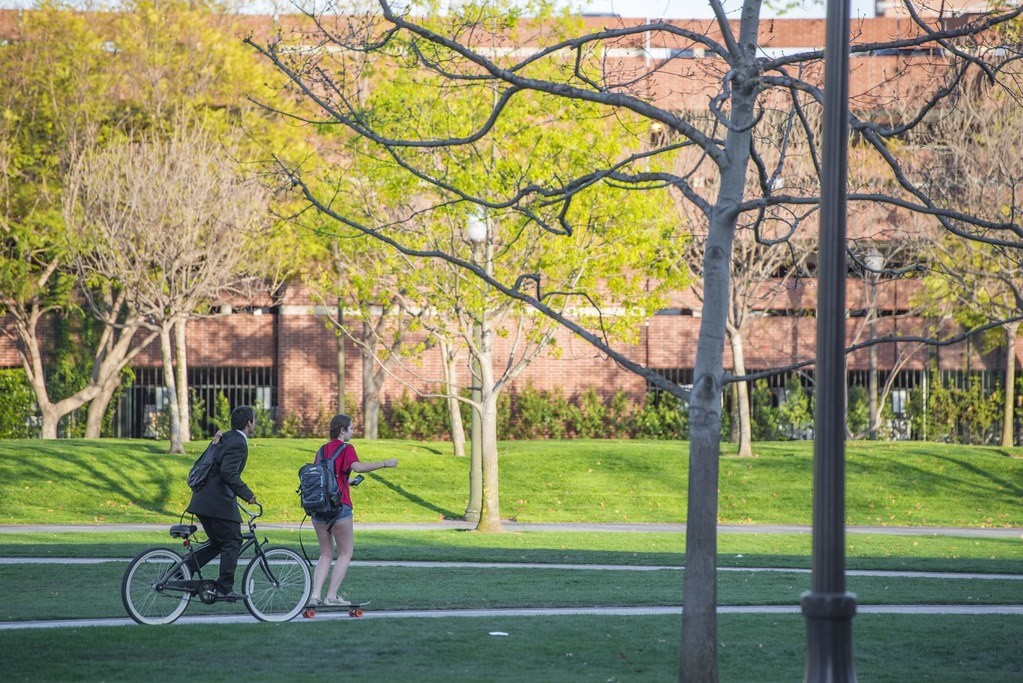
[
  {"xmin": 325, "ymin": 594, "xmax": 352, "ymax": 606},
  {"xmin": 308, "ymin": 597, "xmax": 323, "ymax": 606}
]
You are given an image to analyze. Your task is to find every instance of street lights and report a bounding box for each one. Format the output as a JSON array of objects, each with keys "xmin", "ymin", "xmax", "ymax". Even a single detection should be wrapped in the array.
[
  {"xmin": 462, "ymin": 220, "xmax": 487, "ymax": 522},
  {"xmin": 864, "ymin": 252, "xmax": 883, "ymax": 440}
]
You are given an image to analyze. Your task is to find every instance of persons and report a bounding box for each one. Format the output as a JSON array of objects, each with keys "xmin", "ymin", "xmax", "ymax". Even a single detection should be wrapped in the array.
[
  {"xmin": 172, "ymin": 406, "xmax": 257, "ymax": 600},
  {"xmin": 308, "ymin": 415, "xmax": 398, "ymax": 605}
]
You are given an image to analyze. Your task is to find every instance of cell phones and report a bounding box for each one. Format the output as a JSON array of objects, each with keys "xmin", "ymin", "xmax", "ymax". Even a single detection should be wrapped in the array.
[{"xmin": 351, "ymin": 474, "xmax": 365, "ymax": 486}]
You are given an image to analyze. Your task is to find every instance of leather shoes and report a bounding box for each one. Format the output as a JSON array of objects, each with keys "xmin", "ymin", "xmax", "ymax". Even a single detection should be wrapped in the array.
[{"xmin": 212, "ymin": 589, "xmax": 246, "ymax": 601}]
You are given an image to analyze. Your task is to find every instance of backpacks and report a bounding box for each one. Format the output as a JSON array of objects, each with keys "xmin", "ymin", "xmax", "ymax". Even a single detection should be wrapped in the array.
[
  {"xmin": 186, "ymin": 429, "xmax": 235, "ymax": 492},
  {"xmin": 300, "ymin": 440, "xmax": 351, "ymax": 520}
]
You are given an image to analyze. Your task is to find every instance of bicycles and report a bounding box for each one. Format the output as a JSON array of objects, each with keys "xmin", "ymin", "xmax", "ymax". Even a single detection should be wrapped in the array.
[{"xmin": 120, "ymin": 499, "xmax": 313, "ymax": 625}]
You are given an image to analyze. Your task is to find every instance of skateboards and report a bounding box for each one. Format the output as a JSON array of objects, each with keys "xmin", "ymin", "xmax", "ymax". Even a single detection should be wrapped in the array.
[{"xmin": 290, "ymin": 600, "xmax": 372, "ymax": 618}]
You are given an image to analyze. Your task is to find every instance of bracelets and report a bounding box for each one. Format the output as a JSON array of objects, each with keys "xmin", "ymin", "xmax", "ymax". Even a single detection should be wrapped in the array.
[{"xmin": 383, "ymin": 461, "xmax": 386, "ymax": 468}]
